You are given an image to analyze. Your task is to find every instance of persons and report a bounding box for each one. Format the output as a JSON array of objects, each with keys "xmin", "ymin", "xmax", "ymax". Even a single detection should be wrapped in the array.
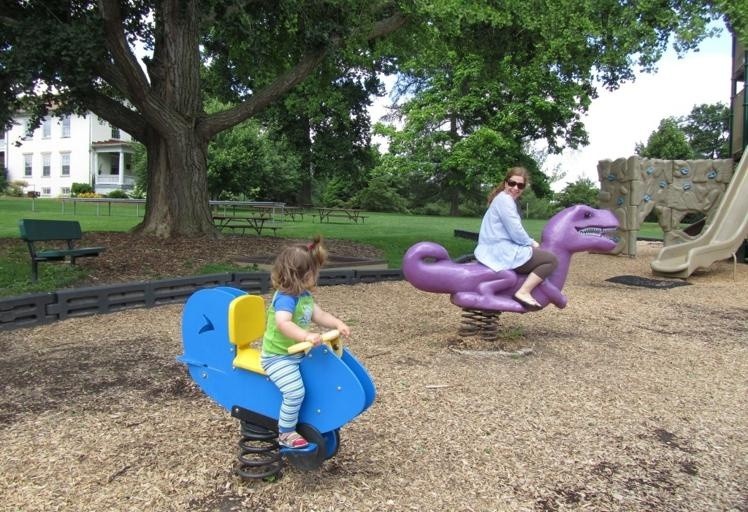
[
  {"xmin": 260, "ymin": 235, "xmax": 350, "ymax": 449},
  {"xmin": 473, "ymin": 166, "xmax": 558, "ymax": 310}
]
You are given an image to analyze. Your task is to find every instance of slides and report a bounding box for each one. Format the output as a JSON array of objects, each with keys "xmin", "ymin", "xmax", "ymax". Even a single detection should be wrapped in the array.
[{"xmin": 648, "ymin": 148, "xmax": 748, "ymax": 278}]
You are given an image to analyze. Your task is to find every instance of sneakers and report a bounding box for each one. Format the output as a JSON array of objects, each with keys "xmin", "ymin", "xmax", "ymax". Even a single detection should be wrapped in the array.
[{"xmin": 276, "ymin": 429, "xmax": 309, "ymax": 449}]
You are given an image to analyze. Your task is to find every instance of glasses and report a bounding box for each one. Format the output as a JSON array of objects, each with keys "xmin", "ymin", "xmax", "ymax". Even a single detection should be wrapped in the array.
[{"xmin": 505, "ymin": 178, "xmax": 527, "ymax": 190}]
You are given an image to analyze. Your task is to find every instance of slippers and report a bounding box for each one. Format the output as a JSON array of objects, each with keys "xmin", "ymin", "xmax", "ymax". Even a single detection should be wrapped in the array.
[{"xmin": 512, "ymin": 294, "xmax": 545, "ymax": 310}]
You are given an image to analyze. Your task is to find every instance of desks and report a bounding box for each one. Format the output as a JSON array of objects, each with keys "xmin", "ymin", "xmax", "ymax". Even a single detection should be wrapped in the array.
[
  {"xmin": 212, "ymin": 213, "xmax": 274, "ymax": 237},
  {"xmin": 251, "ymin": 202, "xmax": 304, "ymax": 222},
  {"xmin": 313, "ymin": 205, "xmax": 365, "ymax": 225}
]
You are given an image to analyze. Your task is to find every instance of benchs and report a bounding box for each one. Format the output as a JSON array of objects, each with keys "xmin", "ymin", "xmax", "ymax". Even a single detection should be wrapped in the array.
[
  {"xmin": 16, "ymin": 216, "xmax": 106, "ymax": 283},
  {"xmin": 312, "ymin": 212, "xmax": 369, "ymax": 224},
  {"xmin": 217, "ymin": 222, "xmax": 283, "ymax": 234}
]
